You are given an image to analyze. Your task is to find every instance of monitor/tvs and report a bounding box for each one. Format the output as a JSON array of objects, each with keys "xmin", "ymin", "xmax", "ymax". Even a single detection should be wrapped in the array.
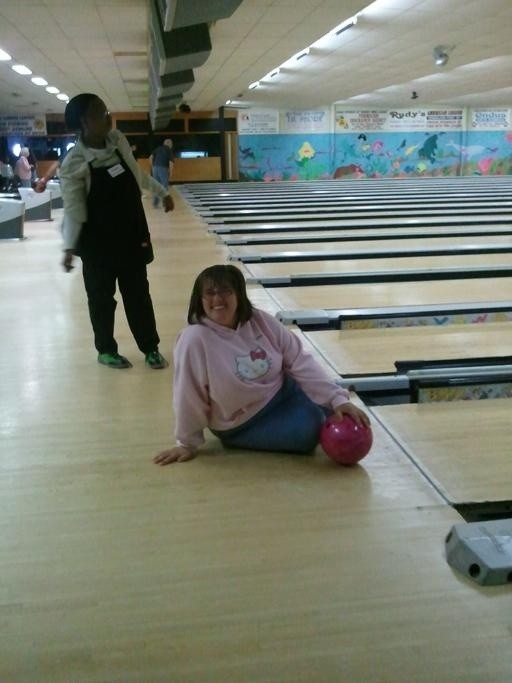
[
  {"xmin": 176, "ymin": 148, "xmax": 208, "ymax": 158},
  {"xmin": 148, "ymin": 0, "xmax": 243, "ymax": 131}
]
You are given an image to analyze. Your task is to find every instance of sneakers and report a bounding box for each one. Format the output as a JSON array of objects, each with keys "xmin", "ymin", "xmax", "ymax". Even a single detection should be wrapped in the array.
[
  {"xmin": 145, "ymin": 348, "xmax": 169, "ymax": 369},
  {"xmin": 98, "ymin": 352, "xmax": 132, "ymax": 369}
]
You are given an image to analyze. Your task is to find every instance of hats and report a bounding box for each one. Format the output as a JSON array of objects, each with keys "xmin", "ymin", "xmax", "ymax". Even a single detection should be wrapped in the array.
[{"xmin": 65, "ymin": 94, "xmax": 98, "ymax": 129}]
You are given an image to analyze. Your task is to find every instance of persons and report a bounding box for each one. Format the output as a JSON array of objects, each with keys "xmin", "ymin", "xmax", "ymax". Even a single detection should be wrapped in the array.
[
  {"xmin": 151, "ymin": 263, "xmax": 370, "ymax": 468},
  {"xmin": 60, "ymin": 91, "xmax": 174, "ymax": 370},
  {"xmin": 149, "ymin": 139, "xmax": 177, "ymax": 208},
  {"xmin": 0, "ymin": 146, "xmax": 36, "ymax": 193},
  {"xmin": 34, "ymin": 134, "xmax": 81, "ymax": 257}
]
